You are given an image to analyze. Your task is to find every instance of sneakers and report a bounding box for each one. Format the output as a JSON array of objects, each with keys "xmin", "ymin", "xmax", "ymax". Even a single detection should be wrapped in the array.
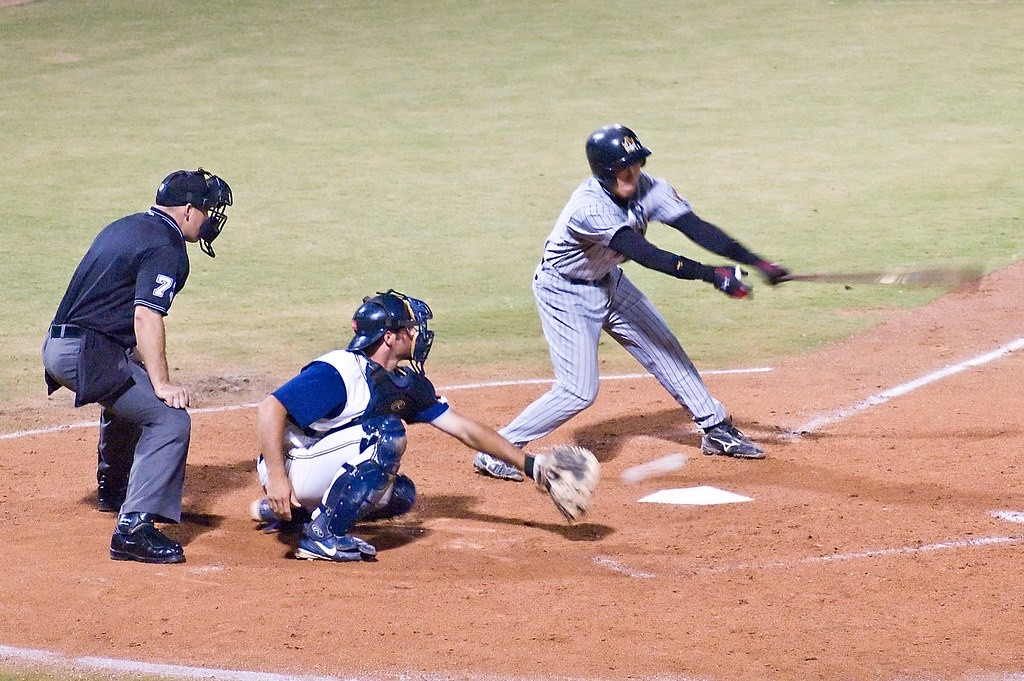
[
  {"xmin": 250, "ymin": 497, "xmax": 278, "ymax": 521},
  {"xmin": 473, "ymin": 452, "xmax": 524, "ymax": 481},
  {"xmin": 291, "ymin": 530, "xmax": 376, "ymax": 562},
  {"xmin": 700, "ymin": 415, "xmax": 766, "ymax": 459}
]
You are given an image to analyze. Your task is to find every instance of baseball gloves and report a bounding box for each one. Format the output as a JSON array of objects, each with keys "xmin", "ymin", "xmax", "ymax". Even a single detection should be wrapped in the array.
[{"xmin": 521, "ymin": 444, "xmax": 603, "ymax": 526}]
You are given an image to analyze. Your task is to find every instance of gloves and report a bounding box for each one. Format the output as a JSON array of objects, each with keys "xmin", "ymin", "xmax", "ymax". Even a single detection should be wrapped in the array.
[
  {"xmin": 754, "ymin": 259, "xmax": 791, "ymax": 287},
  {"xmin": 702, "ymin": 265, "xmax": 754, "ymax": 298}
]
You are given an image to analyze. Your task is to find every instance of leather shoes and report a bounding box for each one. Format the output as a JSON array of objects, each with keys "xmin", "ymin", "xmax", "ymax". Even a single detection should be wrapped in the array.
[
  {"xmin": 111, "ymin": 525, "xmax": 185, "ymax": 562},
  {"xmin": 97, "ymin": 478, "xmax": 128, "ymax": 511}
]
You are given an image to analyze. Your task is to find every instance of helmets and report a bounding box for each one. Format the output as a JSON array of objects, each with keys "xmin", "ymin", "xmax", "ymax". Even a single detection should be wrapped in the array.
[
  {"xmin": 586, "ymin": 124, "xmax": 653, "ymax": 185},
  {"xmin": 345, "ymin": 294, "xmax": 410, "ymax": 352}
]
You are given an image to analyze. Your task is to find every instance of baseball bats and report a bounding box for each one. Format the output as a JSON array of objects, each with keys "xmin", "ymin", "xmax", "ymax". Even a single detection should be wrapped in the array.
[{"xmin": 766, "ymin": 269, "xmax": 980, "ymax": 297}]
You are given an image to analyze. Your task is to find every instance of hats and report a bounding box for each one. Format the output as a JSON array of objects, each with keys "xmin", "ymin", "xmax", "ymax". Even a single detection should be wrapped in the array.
[{"xmin": 156, "ymin": 171, "xmax": 209, "ymax": 206}]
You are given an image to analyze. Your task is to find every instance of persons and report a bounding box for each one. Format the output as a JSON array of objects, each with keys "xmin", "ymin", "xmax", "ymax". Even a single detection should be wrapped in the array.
[
  {"xmin": 249, "ymin": 292, "xmax": 599, "ymax": 561},
  {"xmin": 42, "ymin": 169, "xmax": 233, "ymax": 563},
  {"xmin": 473, "ymin": 122, "xmax": 794, "ymax": 480}
]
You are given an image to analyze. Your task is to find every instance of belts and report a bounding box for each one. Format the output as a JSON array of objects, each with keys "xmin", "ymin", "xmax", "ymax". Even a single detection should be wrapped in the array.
[{"xmin": 50, "ymin": 325, "xmax": 82, "ymax": 338}]
